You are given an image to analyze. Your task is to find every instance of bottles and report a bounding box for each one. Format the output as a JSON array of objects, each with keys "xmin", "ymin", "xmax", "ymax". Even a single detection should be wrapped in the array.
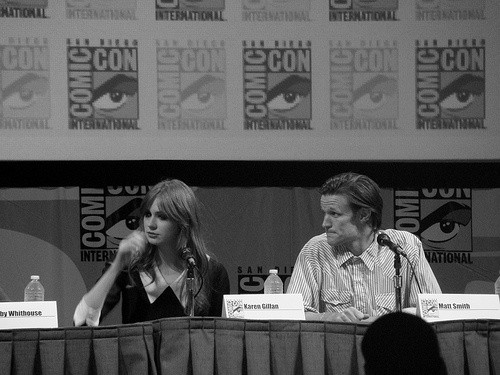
[
  {"xmin": 264, "ymin": 269, "xmax": 283, "ymax": 294},
  {"xmin": 23, "ymin": 275, "xmax": 45, "ymax": 301}
]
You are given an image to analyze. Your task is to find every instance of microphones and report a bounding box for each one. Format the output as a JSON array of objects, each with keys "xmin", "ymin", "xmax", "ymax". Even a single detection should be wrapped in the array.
[
  {"xmin": 377, "ymin": 233, "xmax": 407, "ymax": 257},
  {"xmin": 181, "ymin": 248, "xmax": 195, "ymax": 267}
]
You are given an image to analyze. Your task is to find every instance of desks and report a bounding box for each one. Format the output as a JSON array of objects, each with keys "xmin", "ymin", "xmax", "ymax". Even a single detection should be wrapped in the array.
[{"xmin": 0, "ymin": 317, "xmax": 500, "ymax": 375}]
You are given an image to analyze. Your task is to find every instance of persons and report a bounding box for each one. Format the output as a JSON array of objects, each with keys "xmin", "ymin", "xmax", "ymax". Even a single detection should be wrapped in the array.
[
  {"xmin": 286, "ymin": 173, "xmax": 443, "ymax": 324},
  {"xmin": 73, "ymin": 179, "xmax": 230, "ymax": 327}
]
